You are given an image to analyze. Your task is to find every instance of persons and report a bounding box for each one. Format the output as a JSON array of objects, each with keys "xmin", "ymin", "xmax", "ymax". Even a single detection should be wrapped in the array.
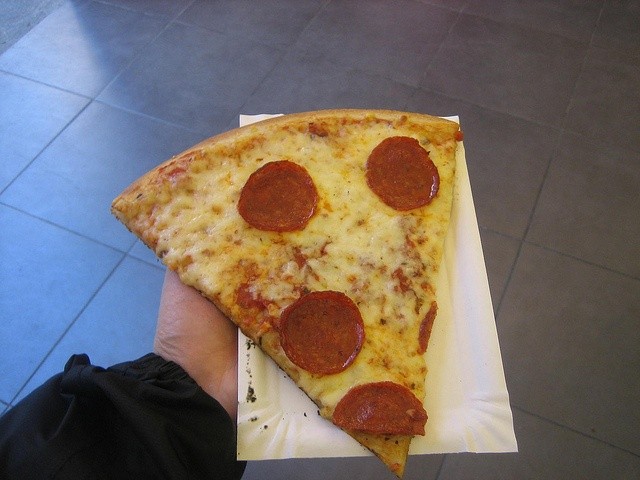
[{"xmin": 0, "ymin": 267, "xmax": 246, "ymax": 479}]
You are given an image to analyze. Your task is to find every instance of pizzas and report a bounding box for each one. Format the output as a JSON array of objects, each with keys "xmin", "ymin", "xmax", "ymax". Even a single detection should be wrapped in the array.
[{"xmin": 111, "ymin": 107, "xmax": 461, "ymax": 475}]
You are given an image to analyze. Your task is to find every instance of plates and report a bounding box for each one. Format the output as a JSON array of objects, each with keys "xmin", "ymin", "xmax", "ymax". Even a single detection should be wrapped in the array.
[{"xmin": 236, "ymin": 112, "xmax": 519, "ymax": 461}]
